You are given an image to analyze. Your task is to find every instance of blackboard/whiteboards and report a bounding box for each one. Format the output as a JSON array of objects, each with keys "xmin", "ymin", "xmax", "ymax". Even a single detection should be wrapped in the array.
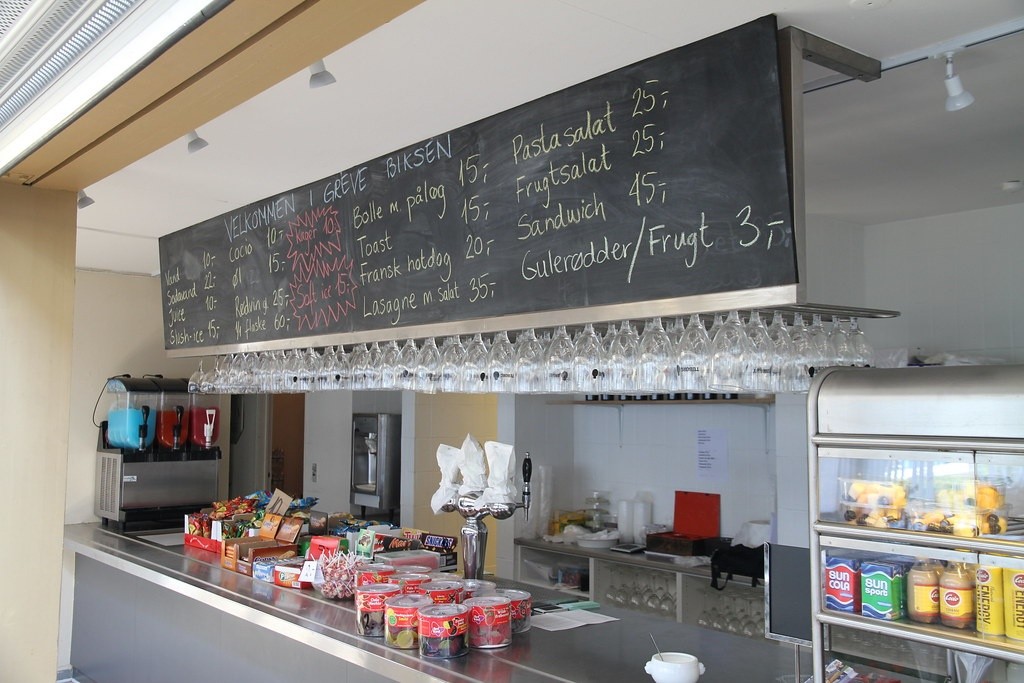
[{"xmin": 154, "ymin": 15, "xmax": 807, "ymax": 358}]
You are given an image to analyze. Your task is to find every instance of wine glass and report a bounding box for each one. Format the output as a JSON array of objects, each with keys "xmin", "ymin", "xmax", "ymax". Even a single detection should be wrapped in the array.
[
  {"xmin": 187, "ymin": 309, "xmax": 875, "ymax": 394},
  {"xmin": 695, "ymin": 582, "xmax": 764, "ymax": 638},
  {"xmin": 603, "ymin": 562, "xmax": 676, "ymax": 613}
]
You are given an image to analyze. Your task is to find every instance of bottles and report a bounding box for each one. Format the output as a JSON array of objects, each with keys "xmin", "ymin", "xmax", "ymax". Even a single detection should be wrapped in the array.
[{"xmin": 907, "ymin": 547, "xmax": 978, "ymax": 632}]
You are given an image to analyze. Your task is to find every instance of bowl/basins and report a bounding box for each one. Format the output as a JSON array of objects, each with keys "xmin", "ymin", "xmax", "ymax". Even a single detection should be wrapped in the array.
[{"xmin": 310, "ymin": 567, "xmax": 355, "ymax": 600}]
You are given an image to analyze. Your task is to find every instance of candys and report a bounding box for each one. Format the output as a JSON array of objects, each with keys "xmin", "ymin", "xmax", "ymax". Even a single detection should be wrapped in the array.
[{"xmin": 309, "ymin": 549, "xmax": 367, "ymax": 598}]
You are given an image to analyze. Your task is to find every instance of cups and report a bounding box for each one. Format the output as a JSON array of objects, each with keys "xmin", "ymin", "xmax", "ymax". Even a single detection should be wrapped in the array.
[
  {"xmin": 617, "ymin": 497, "xmax": 651, "ymax": 544},
  {"xmin": 644, "ymin": 652, "xmax": 705, "ymax": 683}
]
